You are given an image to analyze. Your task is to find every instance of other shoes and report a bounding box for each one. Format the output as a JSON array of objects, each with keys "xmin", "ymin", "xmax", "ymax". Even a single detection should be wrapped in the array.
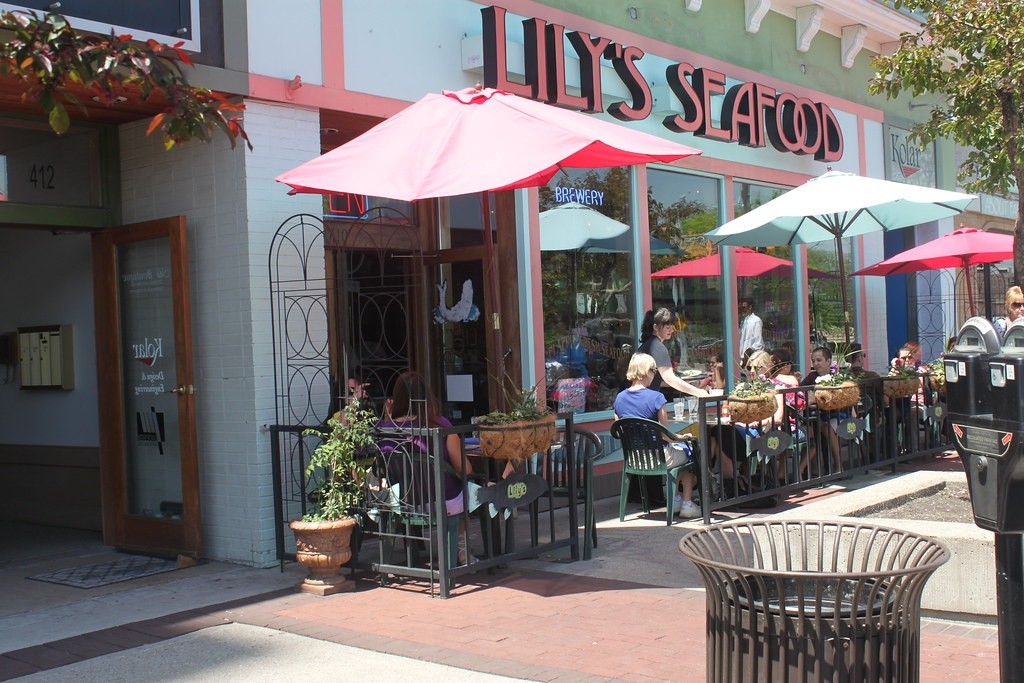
[
  {"xmin": 781, "ymin": 476, "xmax": 794, "ymax": 485},
  {"xmin": 839, "ymin": 474, "xmax": 854, "ymax": 480}
]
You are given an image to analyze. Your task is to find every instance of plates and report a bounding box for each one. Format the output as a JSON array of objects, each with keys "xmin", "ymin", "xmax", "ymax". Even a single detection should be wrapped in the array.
[{"xmin": 683, "ymin": 369, "xmax": 703, "ymax": 377}]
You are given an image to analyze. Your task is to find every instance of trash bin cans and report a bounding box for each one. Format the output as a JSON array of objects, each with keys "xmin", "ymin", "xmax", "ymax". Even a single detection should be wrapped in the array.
[{"xmin": 679, "ymin": 519, "xmax": 952, "ymax": 683}]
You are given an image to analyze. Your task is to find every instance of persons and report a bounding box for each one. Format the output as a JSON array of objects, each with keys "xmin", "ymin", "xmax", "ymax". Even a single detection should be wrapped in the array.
[
  {"xmin": 735, "ymin": 350, "xmax": 817, "ymax": 484},
  {"xmin": 613, "ymin": 353, "xmax": 705, "ymax": 518},
  {"xmin": 844, "ymin": 336, "xmax": 964, "ymax": 455},
  {"xmin": 699, "ymin": 354, "xmax": 726, "ymax": 390},
  {"xmin": 797, "ymin": 348, "xmax": 857, "ymax": 475},
  {"xmin": 344, "ymin": 371, "xmax": 375, "ymax": 419},
  {"xmin": 738, "ymin": 301, "xmax": 766, "ymax": 362},
  {"xmin": 617, "ymin": 307, "xmax": 713, "ymax": 507},
  {"xmin": 992, "ymin": 287, "xmax": 1024, "ymax": 345},
  {"xmin": 380, "ymin": 372, "xmax": 479, "ymax": 565}
]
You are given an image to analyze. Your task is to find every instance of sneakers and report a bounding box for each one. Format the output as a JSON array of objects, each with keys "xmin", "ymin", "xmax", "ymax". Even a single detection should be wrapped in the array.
[
  {"xmin": 674, "ymin": 498, "xmax": 683, "ymax": 513},
  {"xmin": 679, "ymin": 502, "xmax": 702, "ymax": 518}
]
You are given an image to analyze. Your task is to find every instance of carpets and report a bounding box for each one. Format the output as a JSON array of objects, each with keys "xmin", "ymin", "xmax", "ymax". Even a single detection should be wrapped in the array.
[{"xmin": 25, "ymin": 555, "xmax": 209, "ymax": 589}]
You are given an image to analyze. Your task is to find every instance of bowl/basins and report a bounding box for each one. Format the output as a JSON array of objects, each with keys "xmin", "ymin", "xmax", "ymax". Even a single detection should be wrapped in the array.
[{"xmin": 709, "ymin": 388, "xmax": 723, "ymax": 396}]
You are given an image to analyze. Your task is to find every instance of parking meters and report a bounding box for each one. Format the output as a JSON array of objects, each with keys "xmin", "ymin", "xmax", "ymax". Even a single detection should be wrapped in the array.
[{"xmin": 946, "ymin": 315, "xmax": 1024, "ymax": 683}]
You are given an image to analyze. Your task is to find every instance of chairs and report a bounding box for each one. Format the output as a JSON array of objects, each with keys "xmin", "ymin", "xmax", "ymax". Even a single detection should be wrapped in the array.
[{"xmin": 342, "ymin": 392, "xmax": 939, "ymax": 588}]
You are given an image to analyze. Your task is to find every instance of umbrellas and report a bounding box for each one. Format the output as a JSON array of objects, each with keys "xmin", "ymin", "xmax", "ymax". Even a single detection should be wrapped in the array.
[
  {"xmin": 274, "ymin": 83, "xmax": 703, "ymax": 416},
  {"xmin": 539, "ymin": 202, "xmax": 691, "ymax": 328},
  {"xmin": 848, "ymin": 227, "xmax": 1015, "ymax": 318},
  {"xmin": 651, "ymin": 246, "xmax": 839, "ymax": 302},
  {"xmin": 701, "ymin": 171, "xmax": 980, "ymax": 375}
]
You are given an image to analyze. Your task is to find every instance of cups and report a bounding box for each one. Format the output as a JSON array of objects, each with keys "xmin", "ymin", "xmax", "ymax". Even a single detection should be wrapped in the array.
[
  {"xmin": 687, "ymin": 397, "xmax": 698, "ymax": 416},
  {"xmin": 673, "ymin": 398, "xmax": 685, "ymax": 418}
]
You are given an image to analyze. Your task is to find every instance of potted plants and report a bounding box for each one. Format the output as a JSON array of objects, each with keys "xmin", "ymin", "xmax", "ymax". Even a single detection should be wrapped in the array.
[
  {"xmin": 289, "ymin": 400, "xmax": 373, "ymax": 597},
  {"xmin": 0, "ymin": 8, "xmax": 257, "ymax": 152},
  {"xmin": 475, "ymin": 360, "xmax": 566, "ymax": 461}
]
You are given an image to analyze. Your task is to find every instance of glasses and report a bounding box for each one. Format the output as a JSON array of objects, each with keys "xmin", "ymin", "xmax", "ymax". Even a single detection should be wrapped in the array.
[
  {"xmin": 650, "ymin": 368, "xmax": 657, "ymax": 374},
  {"xmin": 1011, "ymin": 302, "xmax": 1024, "ymax": 309},
  {"xmin": 747, "ymin": 366, "xmax": 759, "ymax": 371},
  {"xmin": 856, "ymin": 352, "xmax": 866, "ymax": 358},
  {"xmin": 349, "ymin": 388, "xmax": 355, "ymax": 395},
  {"xmin": 901, "ymin": 356, "xmax": 912, "ymax": 359}
]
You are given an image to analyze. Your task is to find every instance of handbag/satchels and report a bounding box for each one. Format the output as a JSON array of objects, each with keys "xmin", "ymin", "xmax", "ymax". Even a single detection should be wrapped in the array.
[{"xmin": 724, "ymin": 475, "xmax": 776, "ymax": 508}]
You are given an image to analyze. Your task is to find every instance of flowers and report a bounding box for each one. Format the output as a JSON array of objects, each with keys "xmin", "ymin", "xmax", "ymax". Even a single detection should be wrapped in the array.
[
  {"xmin": 815, "ymin": 339, "xmax": 875, "ymax": 388},
  {"xmin": 728, "ymin": 353, "xmax": 790, "ymax": 399},
  {"xmin": 893, "ymin": 360, "xmax": 920, "ymax": 380}
]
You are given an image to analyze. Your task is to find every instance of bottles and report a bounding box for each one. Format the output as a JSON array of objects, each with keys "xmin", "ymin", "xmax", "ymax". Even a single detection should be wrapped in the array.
[{"xmin": 721, "ymin": 401, "xmax": 729, "ymax": 417}]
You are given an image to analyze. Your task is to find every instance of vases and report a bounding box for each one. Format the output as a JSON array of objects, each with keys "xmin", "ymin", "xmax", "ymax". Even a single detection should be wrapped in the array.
[
  {"xmin": 728, "ymin": 394, "xmax": 777, "ymax": 422},
  {"xmin": 815, "ymin": 383, "xmax": 859, "ymax": 411},
  {"xmin": 882, "ymin": 377, "xmax": 920, "ymax": 398}
]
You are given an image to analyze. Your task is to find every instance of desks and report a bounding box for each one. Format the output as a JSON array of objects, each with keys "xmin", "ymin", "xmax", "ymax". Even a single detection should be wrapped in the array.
[{"xmin": 671, "ymin": 413, "xmax": 732, "ymax": 427}]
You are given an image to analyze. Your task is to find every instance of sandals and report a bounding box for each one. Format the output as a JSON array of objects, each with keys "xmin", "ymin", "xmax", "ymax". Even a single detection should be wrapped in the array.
[{"xmin": 459, "ymin": 531, "xmax": 480, "ymax": 565}]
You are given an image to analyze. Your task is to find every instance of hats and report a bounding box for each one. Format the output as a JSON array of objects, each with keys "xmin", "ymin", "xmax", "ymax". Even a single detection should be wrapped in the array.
[{"xmin": 844, "ymin": 343, "xmax": 862, "ymax": 363}]
